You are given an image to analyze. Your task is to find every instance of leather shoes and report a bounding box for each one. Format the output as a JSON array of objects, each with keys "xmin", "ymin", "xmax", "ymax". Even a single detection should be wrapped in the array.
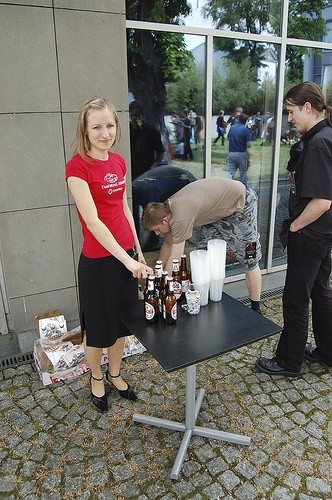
[
  {"xmin": 255, "ymin": 357, "xmax": 301, "ymax": 377},
  {"xmin": 303, "ymin": 348, "xmax": 332, "ymax": 365}
]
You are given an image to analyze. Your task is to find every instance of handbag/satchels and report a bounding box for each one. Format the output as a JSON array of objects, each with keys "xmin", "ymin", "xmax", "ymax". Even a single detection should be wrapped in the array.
[{"xmin": 218, "ymin": 127, "xmax": 224, "ymax": 133}]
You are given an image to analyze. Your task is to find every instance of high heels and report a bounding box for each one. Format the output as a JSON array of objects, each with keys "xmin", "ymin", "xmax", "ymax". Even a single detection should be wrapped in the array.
[
  {"xmin": 89, "ymin": 372, "xmax": 109, "ymax": 412},
  {"xmin": 106, "ymin": 369, "xmax": 138, "ymax": 401}
]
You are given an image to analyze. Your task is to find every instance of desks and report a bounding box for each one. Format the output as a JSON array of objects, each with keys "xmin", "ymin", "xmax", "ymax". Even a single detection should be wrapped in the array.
[{"xmin": 116, "ymin": 292, "xmax": 283, "ymax": 482}]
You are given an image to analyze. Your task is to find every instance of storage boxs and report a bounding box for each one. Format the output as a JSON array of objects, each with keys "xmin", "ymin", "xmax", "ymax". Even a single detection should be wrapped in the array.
[{"xmin": 32, "ymin": 310, "xmax": 148, "ymax": 386}]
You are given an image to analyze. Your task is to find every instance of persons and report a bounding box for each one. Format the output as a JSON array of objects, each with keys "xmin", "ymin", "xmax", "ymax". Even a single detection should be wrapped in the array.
[
  {"xmin": 63, "ymin": 96, "xmax": 153, "ymax": 411},
  {"xmin": 142, "ymin": 177, "xmax": 262, "ymax": 316},
  {"xmin": 130, "ymin": 107, "xmax": 299, "ymax": 252},
  {"xmin": 254, "ymin": 81, "xmax": 331, "ymax": 376}
]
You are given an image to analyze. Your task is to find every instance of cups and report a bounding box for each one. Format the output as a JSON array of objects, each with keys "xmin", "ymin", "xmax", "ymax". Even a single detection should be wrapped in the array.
[{"xmin": 184, "ymin": 239, "xmax": 226, "ymax": 314}]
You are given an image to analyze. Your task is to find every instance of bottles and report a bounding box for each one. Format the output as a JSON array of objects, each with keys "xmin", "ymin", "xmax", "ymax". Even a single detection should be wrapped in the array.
[{"xmin": 143, "ymin": 254, "xmax": 191, "ymax": 325}]
